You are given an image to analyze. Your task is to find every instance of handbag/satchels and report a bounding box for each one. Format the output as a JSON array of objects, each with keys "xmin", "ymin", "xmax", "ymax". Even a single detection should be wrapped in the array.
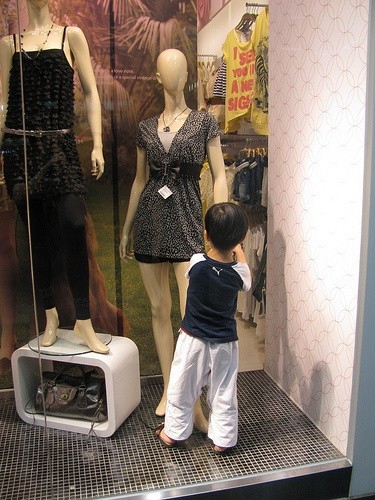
[{"xmin": 25, "ymin": 364, "xmax": 108, "ymax": 423}]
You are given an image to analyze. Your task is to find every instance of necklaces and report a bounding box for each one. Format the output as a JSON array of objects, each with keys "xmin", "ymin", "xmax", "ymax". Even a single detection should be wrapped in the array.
[
  {"xmin": 21, "ymin": 21, "xmax": 55, "ymax": 60},
  {"xmin": 163, "ymin": 107, "xmax": 189, "ymax": 132}
]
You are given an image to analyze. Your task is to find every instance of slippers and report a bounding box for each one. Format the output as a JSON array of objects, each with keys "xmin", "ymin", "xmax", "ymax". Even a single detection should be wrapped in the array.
[
  {"xmin": 210, "ymin": 439, "xmax": 228, "ymax": 454},
  {"xmin": 154, "ymin": 424, "xmax": 177, "ymax": 448}
]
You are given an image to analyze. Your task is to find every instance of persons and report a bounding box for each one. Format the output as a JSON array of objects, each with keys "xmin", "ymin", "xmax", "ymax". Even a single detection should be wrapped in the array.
[
  {"xmin": 119, "ymin": 48, "xmax": 229, "ymax": 433},
  {"xmin": 0, "ymin": 0, "xmax": 110, "ymax": 354},
  {"xmin": 154, "ymin": 201, "xmax": 252, "ymax": 455}
]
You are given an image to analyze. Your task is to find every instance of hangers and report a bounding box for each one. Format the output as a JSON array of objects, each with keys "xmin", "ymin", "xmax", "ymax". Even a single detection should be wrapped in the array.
[
  {"xmin": 230, "ymin": 3, "xmax": 260, "ymax": 31},
  {"xmin": 239, "ymin": 139, "xmax": 267, "ymax": 157},
  {"xmin": 245, "ymin": 205, "xmax": 267, "ymax": 233}
]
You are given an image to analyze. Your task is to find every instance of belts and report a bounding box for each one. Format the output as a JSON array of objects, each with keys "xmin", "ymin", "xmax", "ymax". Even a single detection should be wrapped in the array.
[{"xmin": 1, "ymin": 128, "xmax": 71, "ymax": 138}]
